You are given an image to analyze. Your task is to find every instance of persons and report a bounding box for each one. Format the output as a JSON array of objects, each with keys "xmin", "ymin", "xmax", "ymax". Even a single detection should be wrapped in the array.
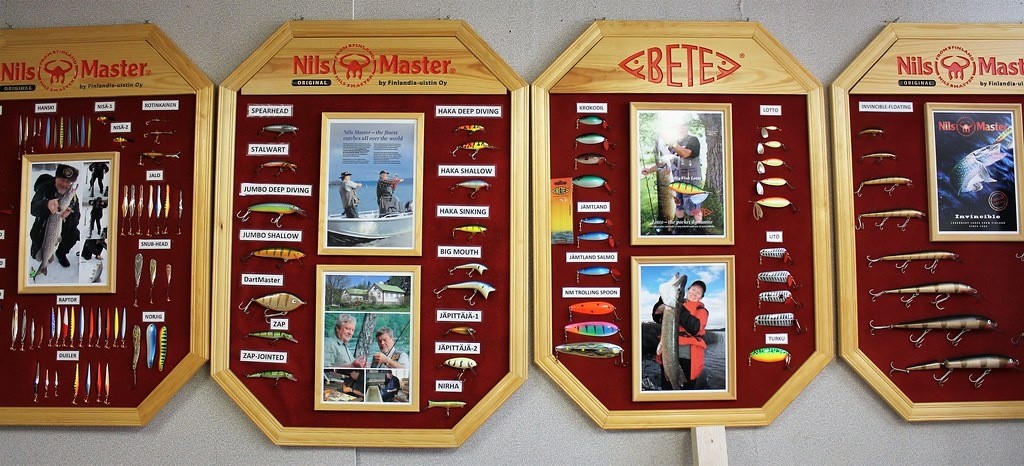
[
  {"xmin": 29, "ymin": 160, "xmax": 108, "ymax": 283},
  {"xmin": 384, "ymin": 370, "xmax": 400, "ymax": 401},
  {"xmin": 339, "ymin": 170, "xmax": 363, "ymax": 218},
  {"xmin": 651, "ymin": 280, "xmax": 710, "ymax": 389},
  {"xmin": 377, "ymin": 170, "xmax": 405, "ymax": 206},
  {"xmin": 640, "ymin": 113, "xmax": 706, "ymax": 235},
  {"xmin": 371, "ymin": 327, "xmax": 408, "ymax": 368},
  {"xmin": 324, "ymin": 314, "xmax": 369, "ymax": 368},
  {"xmin": 343, "ymin": 370, "xmax": 364, "ymax": 398}
]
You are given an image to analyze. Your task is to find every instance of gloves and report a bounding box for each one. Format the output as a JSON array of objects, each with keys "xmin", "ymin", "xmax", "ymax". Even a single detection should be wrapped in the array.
[
  {"xmin": 679, "ymin": 305, "xmax": 700, "ymax": 336},
  {"xmin": 652, "ymin": 295, "xmax": 664, "ymax": 325}
]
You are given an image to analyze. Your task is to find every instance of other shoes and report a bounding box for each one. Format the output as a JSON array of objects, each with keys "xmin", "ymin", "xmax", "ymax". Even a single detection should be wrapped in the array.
[{"xmin": 56, "ymin": 253, "xmax": 70, "ymax": 267}]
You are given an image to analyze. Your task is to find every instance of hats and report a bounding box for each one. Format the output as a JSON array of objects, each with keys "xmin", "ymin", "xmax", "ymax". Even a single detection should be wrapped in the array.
[
  {"xmin": 55, "ymin": 164, "xmax": 79, "ymax": 182},
  {"xmin": 339, "ymin": 171, "xmax": 353, "ymax": 178},
  {"xmin": 379, "ymin": 171, "xmax": 389, "ymax": 174},
  {"xmin": 688, "ymin": 280, "xmax": 706, "ymax": 293}
]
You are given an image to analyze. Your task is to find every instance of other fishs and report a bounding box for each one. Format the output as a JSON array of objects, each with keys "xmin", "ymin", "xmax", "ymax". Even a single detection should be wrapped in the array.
[
  {"xmin": 661, "ymin": 271, "xmax": 688, "ymax": 389},
  {"xmin": 655, "ymin": 139, "xmax": 676, "ymax": 230},
  {"xmin": 89, "ymin": 261, "xmax": 102, "ymax": 283},
  {"xmin": 32, "ymin": 182, "xmax": 78, "ymax": 277},
  {"xmin": 950, "ymin": 143, "xmax": 1007, "ymax": 196}
]
